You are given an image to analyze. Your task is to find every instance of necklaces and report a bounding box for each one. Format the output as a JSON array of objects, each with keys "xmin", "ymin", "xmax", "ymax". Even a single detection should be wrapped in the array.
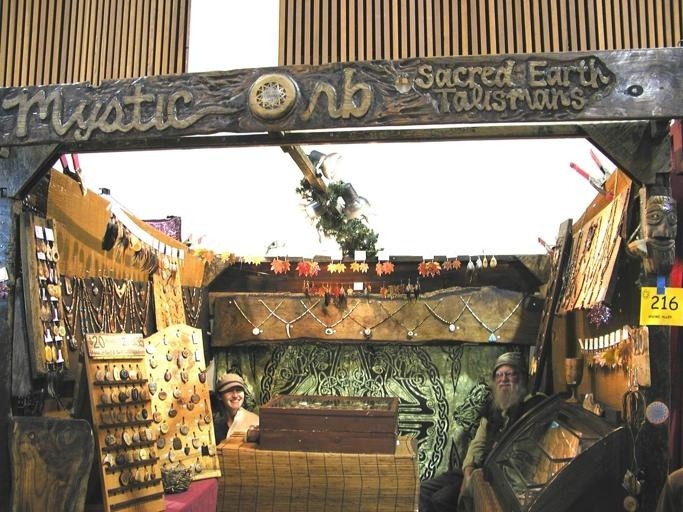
[
  {"xmin": 63, "ymin": 276, "xmax": 154, "ymax": 348},
  {"xmin": 554, "ymin": 203, "xmax": 620, "ymax": 316}
]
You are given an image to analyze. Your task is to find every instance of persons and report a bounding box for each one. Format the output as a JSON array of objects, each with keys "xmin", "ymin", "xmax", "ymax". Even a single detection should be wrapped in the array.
[
  {"xmin": 420, "ymin": 349, "xmax": 540, "ymax": 512},
  {"xmin": 214, "ymin": 371, "xmax": 260, "ymax": 440}
]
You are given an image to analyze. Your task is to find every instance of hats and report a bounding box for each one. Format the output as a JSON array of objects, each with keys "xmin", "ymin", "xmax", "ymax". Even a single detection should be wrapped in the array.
[
  {"xmin": 215, "ymin": 374, "xmax": 246, "ymax": 393},
  {"xmin": 493, "ymin": 352, "xmax": 525, "ymax": 378}
]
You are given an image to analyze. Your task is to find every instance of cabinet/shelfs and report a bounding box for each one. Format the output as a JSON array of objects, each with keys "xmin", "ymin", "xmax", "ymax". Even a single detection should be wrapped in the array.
[{"xmin": 484, "ymin": 390, "xmax": 628, "ymax": 512}]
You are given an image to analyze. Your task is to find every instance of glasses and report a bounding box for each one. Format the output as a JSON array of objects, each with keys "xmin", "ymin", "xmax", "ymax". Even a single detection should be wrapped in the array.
[{"xmin": 495, "ymin": 371, "xmax": 517, "ymax": 377}]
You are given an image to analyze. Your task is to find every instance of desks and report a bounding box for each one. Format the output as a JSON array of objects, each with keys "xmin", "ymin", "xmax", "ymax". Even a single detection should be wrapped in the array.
[
  {"xmin": 159, "ymin": 477, "xmax": 218, "ymax": 511},
  {"xmin": 218, "ymin": 435, "xmax": 419, "ymax": 511},
  {"xmin": 470, "ymin": 469, "xmax": 500, "ymax": 512}
]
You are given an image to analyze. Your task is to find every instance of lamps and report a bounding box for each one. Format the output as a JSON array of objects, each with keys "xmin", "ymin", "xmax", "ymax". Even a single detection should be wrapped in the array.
[{"xmin": 301, "ymin": 149, "xmax": 373, "ymax": 224}]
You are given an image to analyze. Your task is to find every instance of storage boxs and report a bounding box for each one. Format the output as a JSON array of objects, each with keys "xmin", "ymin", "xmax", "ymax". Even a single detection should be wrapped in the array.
[{"xmin": 257, "ymin": 392, "xmax": 399, "ymax": 454}]
